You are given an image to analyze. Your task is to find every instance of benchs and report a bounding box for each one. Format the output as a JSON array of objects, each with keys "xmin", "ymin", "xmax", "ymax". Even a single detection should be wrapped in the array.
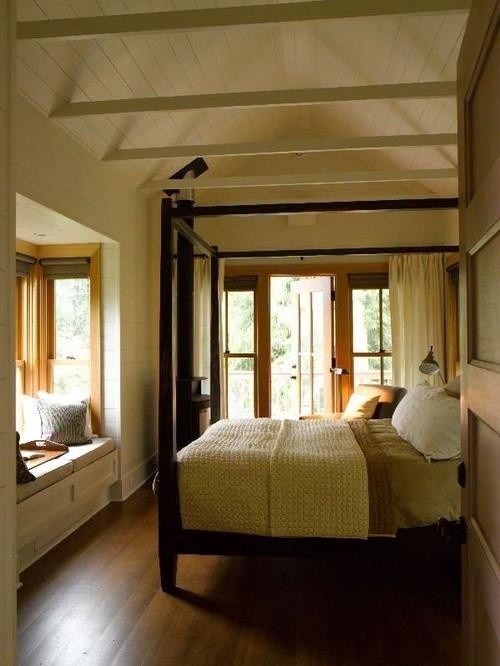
[{"xmin": 16, "ymin": 434, "xmax": 119, "ymax": 577}]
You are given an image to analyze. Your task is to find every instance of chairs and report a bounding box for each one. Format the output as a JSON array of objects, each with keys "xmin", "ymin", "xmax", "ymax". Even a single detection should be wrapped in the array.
[{"xmin": 298, "ymin": 382, "xmax": 407, "ymax": 423}]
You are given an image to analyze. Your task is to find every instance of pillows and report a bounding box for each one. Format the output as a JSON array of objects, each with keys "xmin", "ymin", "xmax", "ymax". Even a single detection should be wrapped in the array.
[
  {"xmin": 391, "ymin": 380, "xmax": 465, "ymax": 465},
  {"xmin": 341, "ymin": 392, "xmax": 380, "ymax": 423},
  {"xmin": 17, "ymin": 387, "xmax": 97, "ymax": 446}
]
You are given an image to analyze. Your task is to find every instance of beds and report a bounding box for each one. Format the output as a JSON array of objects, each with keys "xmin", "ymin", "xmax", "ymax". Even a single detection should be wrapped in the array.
[{"xmin": 151, "ymin": 415, "xmax": 466, "ymax": 594}]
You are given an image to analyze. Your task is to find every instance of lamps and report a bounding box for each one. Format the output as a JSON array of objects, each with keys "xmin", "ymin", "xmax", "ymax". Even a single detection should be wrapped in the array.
[{"xmin": 419, "ymin": 344, "xmax": 447, "ymax": 386}]
